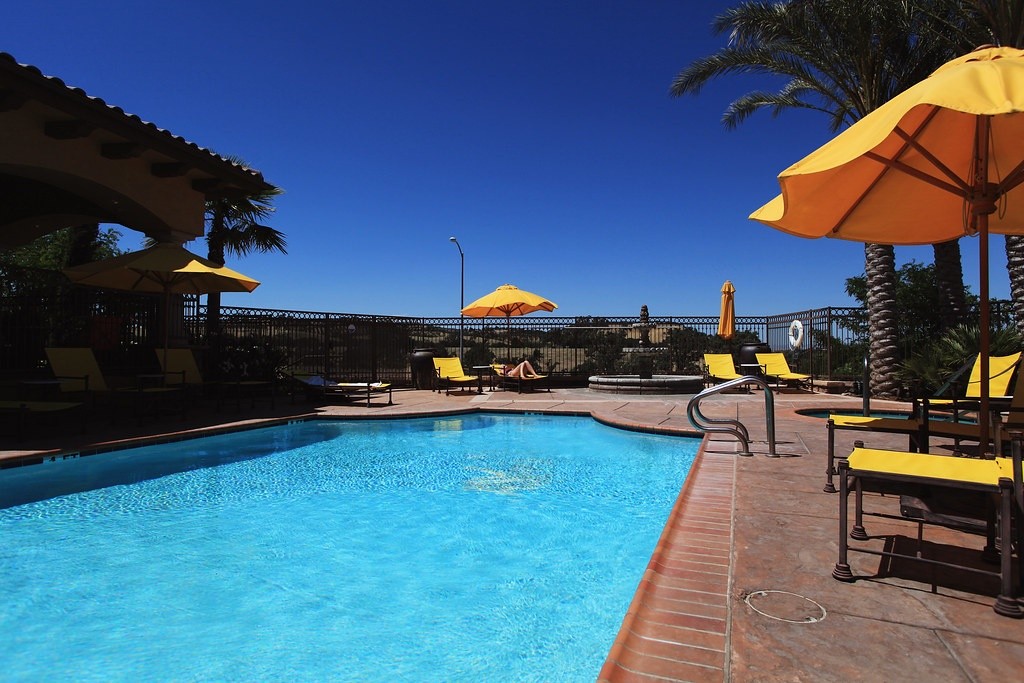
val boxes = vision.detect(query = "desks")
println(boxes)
[739,363,767,390]
[136,370,185,417]
[470,365,497,391]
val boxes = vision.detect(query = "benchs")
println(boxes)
[489,362,552,394]
[918,351,1022,457]
[44,346,185,425]
[755,352,815,395]
[156,347,276,416]
[431,357,483,396]
[0,384,88,443]
[704,353,754,394]
[291,371,393,408]
[838,441,1024,619]
[824,356,1024,493]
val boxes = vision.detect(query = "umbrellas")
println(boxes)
[460,283,558,363]
[62,242,262,388]
[717,280,736,353]
[746,45,1024,457]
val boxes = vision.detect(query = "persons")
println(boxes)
[498,361,542,379]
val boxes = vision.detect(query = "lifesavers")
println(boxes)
[788,320,803,347]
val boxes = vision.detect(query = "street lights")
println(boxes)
[449,236,464,366]
